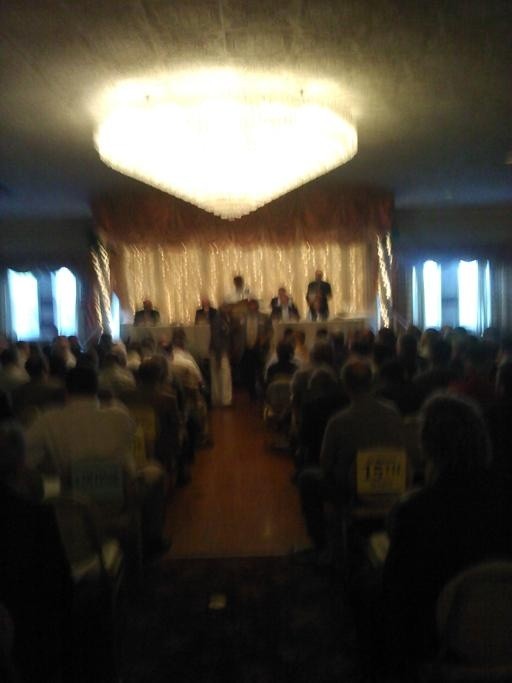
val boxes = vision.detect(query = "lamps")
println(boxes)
[93,66,358,224]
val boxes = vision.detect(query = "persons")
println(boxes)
[270,296,300,321]
[194,299,210,325]
[226,301,273,407]
[222,276,255,316]
[270,289,285,309]
[134,301,159,324]
[305,271,332,320]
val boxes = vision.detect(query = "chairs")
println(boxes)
[336,441,414,570]
[435,562,511,681]
[69,451,147,565]
[37,492,130,681]
